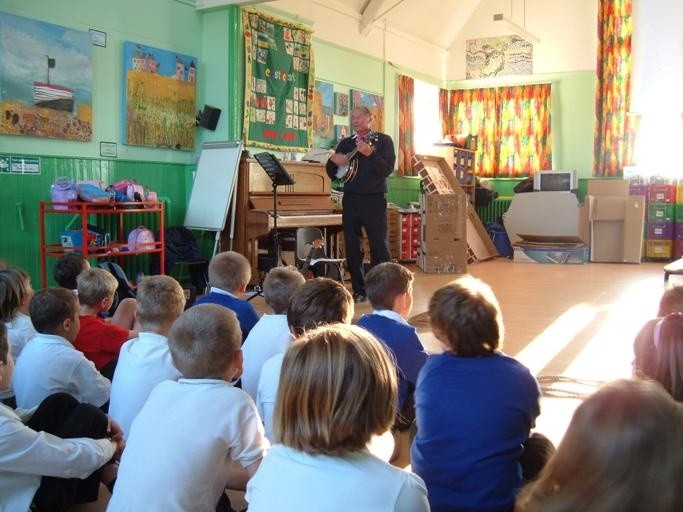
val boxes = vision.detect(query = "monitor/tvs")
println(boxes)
[533,169,578,192]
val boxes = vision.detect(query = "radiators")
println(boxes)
[474,196,514,224]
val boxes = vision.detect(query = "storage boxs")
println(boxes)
[645,183,683,259]
[584,180,645,265]
[425,193,467,273]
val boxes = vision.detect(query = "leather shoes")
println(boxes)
[352,291,367,304]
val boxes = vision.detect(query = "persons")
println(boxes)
[355,261,425,432]
[105,303,269,512]
[241,267,306,399]
[632,313,681,397]
[53,253,90,290]
[0,318,127,511]
[410,274,557,511]
[327,105,396,306]
[191,251,260,341]
[1,266,39,363]
[74,267,140,379]
[520,379,683,512]
[245,323,428,511]
[12,289,112,409]
[109,275,186,436]
[258,277,355,446]
[657,287,682,315]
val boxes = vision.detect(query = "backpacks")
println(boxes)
[99,262,136,316]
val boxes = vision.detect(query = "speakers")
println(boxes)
[195,104,221,131]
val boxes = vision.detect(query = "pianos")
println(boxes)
[218,158,346,286]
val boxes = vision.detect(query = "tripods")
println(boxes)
[244,183,279,301]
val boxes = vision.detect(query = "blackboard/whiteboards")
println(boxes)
[183,141,244,231]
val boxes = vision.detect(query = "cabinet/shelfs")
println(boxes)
[432,145,475,210]
[35,196,170,290]
